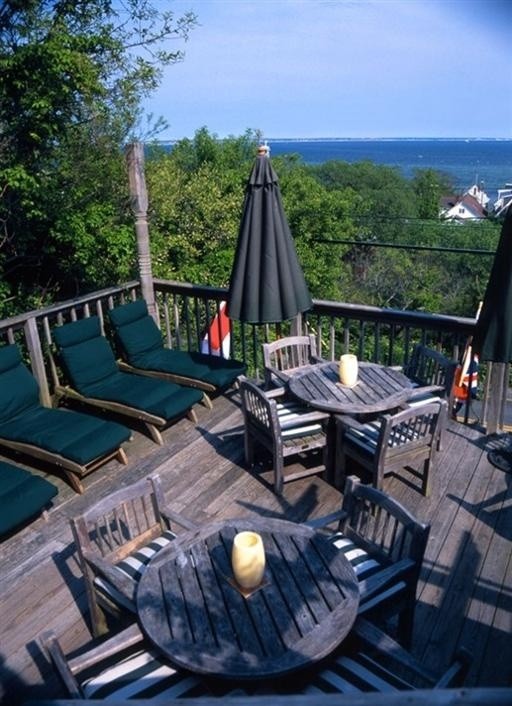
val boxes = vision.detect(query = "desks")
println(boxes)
[288,360,413,468]
[137,515,360,697]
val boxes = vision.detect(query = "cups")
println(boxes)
[338,353,358,387]
[231,530,266,590]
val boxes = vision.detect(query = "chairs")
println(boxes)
[69,472,200,632]
[37,621,247,699]
[298,474,432,651]
[283,616,481,697]
[333,396,447,497]
[108,300,249,410]
[238,378,332,496]
[45,314,204,446]
[0,460,58,539]
[394,341,461,452]
[262,332,328,401]
[0,344,134,496]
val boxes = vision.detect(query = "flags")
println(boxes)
[200,297,232,363]
[451,335,480,414]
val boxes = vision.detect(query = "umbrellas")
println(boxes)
[221,140,321,343]
[469,203,511,366]
